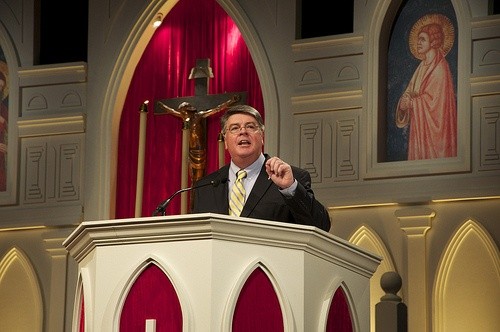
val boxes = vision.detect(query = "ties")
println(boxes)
[228,170,247,218]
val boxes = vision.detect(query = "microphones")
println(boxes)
[221,178,231,185]
[159,178,219,216]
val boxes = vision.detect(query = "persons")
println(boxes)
[156,94,241,215]
[190,103,333,233]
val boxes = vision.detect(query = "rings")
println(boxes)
[281,159,284,164]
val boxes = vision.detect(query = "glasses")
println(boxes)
[225,124,262,134]
